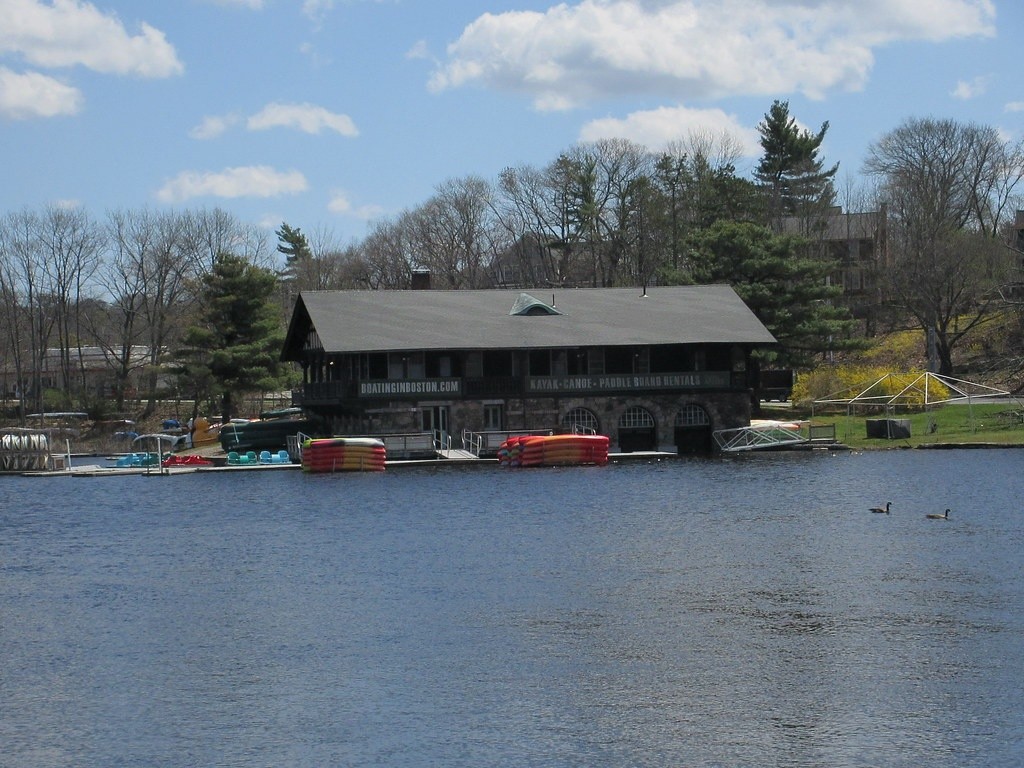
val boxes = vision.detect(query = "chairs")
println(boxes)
[230,451,256,463]
[260,451,289,463]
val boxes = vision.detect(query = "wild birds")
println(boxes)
[926,509,950,519]
[868,501,892,513]
